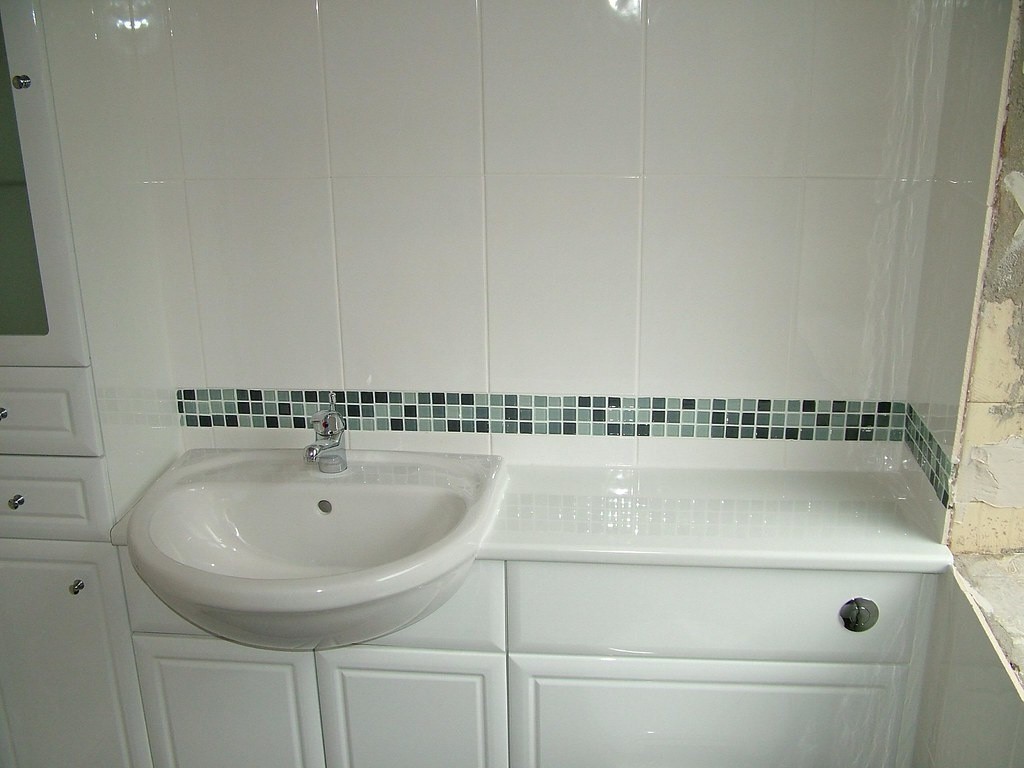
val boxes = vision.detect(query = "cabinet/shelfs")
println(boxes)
[0,0,178,768]
[504,559,938,767]
[118,545,509,768]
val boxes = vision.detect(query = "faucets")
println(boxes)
[303,411,348,473]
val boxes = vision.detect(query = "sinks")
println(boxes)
[127,448,505,651]
